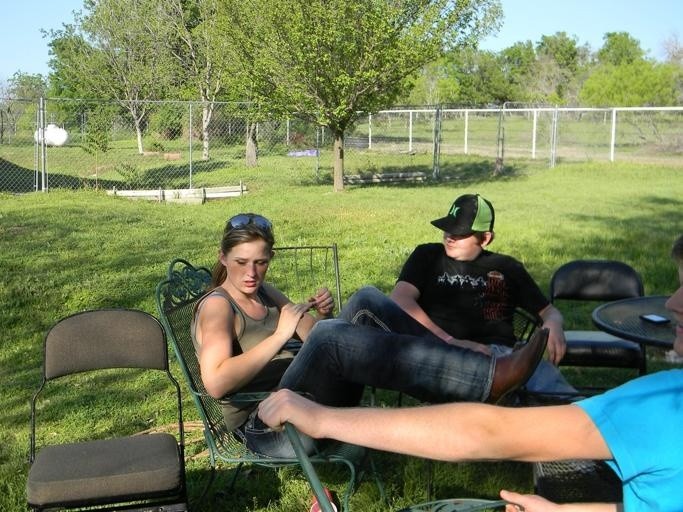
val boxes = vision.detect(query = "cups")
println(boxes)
[309,486,338,512]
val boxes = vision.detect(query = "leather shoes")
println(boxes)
[486,326,552,405]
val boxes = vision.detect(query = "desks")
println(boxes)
[591,296,678,377]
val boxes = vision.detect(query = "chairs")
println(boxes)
[157,258,388,511]
[546,258,647,399]
[24,308,188,511]
[263,243,376,409]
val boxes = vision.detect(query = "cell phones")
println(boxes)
[639,312,671,325]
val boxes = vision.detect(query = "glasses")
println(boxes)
[222,214,273,241]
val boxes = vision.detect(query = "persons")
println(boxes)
[189,212,552,461]
[256,232,683,511]
[384,193,589,406]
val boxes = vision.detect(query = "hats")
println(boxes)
[430,193,494,233]
